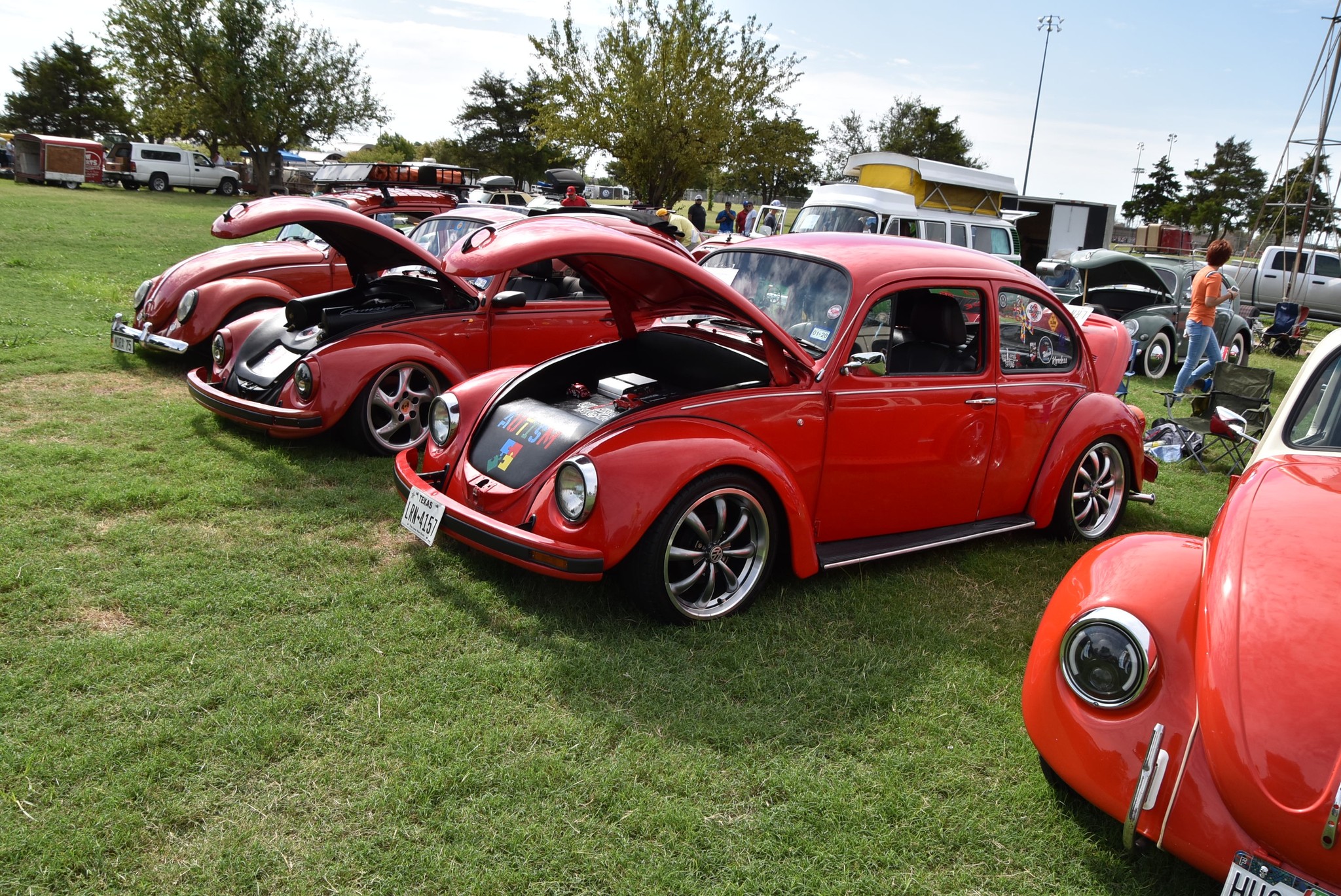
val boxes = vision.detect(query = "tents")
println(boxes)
[239,147,306,162]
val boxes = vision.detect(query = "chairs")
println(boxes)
[1249,301,1311,359]
[1152,360,1273,477]
[883,294,979,374]
[504,258,559,301]
[1239,304,1261,329]
[567,272,604,299]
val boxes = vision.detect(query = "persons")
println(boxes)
[5,138,14,167]
[655,208,707,252]
[688,195,707,232]
[744,202,757,237]
[211,151,225,165]
[102,147,108,160]
[735,201,749,234]
[561,186,586,206]
[225,156,232,165]
[715,201,736,234]
[763,199,781,235]
[1173,239,1238,402]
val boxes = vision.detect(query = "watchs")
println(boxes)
[750,229,752,232]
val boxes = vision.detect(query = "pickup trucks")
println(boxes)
[1196,246,1341,327]
[104,141,241,197]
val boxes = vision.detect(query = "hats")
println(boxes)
[566,186,575,195]
[747,202,754,209]
[743,201,749,206]
[656,208,669,217]
[771,199,785,207]
[725,201,731,206]
[696,195,702,200]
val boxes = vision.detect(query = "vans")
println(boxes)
[750,184,1022,276]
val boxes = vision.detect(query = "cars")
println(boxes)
[691,234,753,262]
[186,197,710,460]
[461,175,533,207]
[1067,248,1252,382]
[392,213,1159,626]
[1023,323,1340,896]
[111,188,460,370]
[526,168,590,208]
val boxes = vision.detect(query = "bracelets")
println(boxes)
[1229,292,1233,299]
